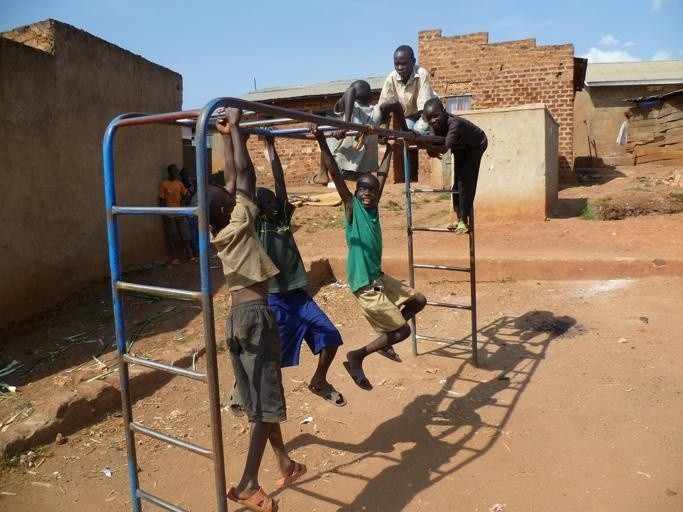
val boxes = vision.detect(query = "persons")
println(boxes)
[309,78,420,186]
[376,43,438,136]
[228,125,346,416]
[189,104,306,512]
[310,124,427,391]
[158,164,196,267]
[424,99,488,234]
[179,167,197,261]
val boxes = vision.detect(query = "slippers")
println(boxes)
[309,384,346,407]
[230,381,245,417]
[343,362,372,390]
[277,461,307,484]
[448,219,469,235]
[227,486,278,512]
[305,175,325,186]
[377,348,401,362]
